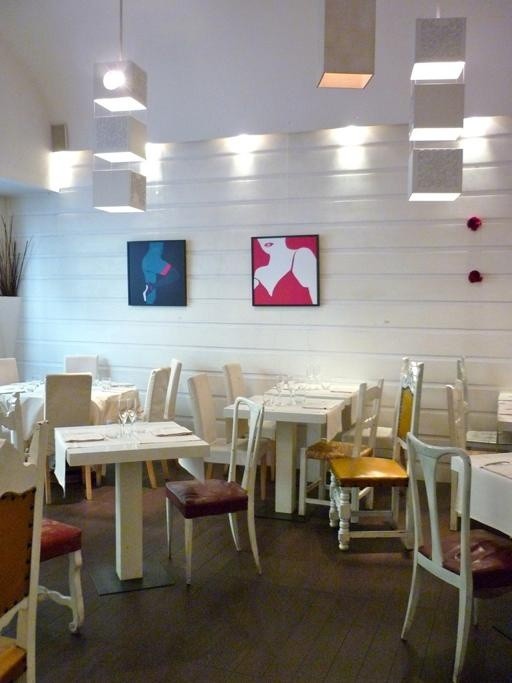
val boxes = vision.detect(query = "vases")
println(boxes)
[0,296,22,358]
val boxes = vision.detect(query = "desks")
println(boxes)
[2,376,142,499]
[448,452,511,629]
[222,378,362,522]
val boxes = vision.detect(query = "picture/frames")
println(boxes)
[126,239,187,307]
[251,234,320,306]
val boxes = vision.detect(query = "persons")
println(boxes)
[141,241,180,306]
[253,238,317,304]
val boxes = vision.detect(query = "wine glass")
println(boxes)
[267,365,323,405]
[117,392,138,439]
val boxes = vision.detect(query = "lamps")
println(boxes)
[406,0,465,203]
[91,0,148,214]
[315,0,374,90]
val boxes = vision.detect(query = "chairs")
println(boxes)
[95,365,170,488]
[62,355,109,477]
[0,418,50,683]
[163,396,266,587]
[1,357,20,385]
[39,515,84,634]
[446,357,512,533]
[328,354,425,552]
[188,371,269,499]
[221,360,277,486]
[44,372,94,506]
[400,433,511,682]
[163,359,183,420]
[297,376,388,518]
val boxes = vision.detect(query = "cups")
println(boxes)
[262,391,275,406]
[103,415,120,437]
